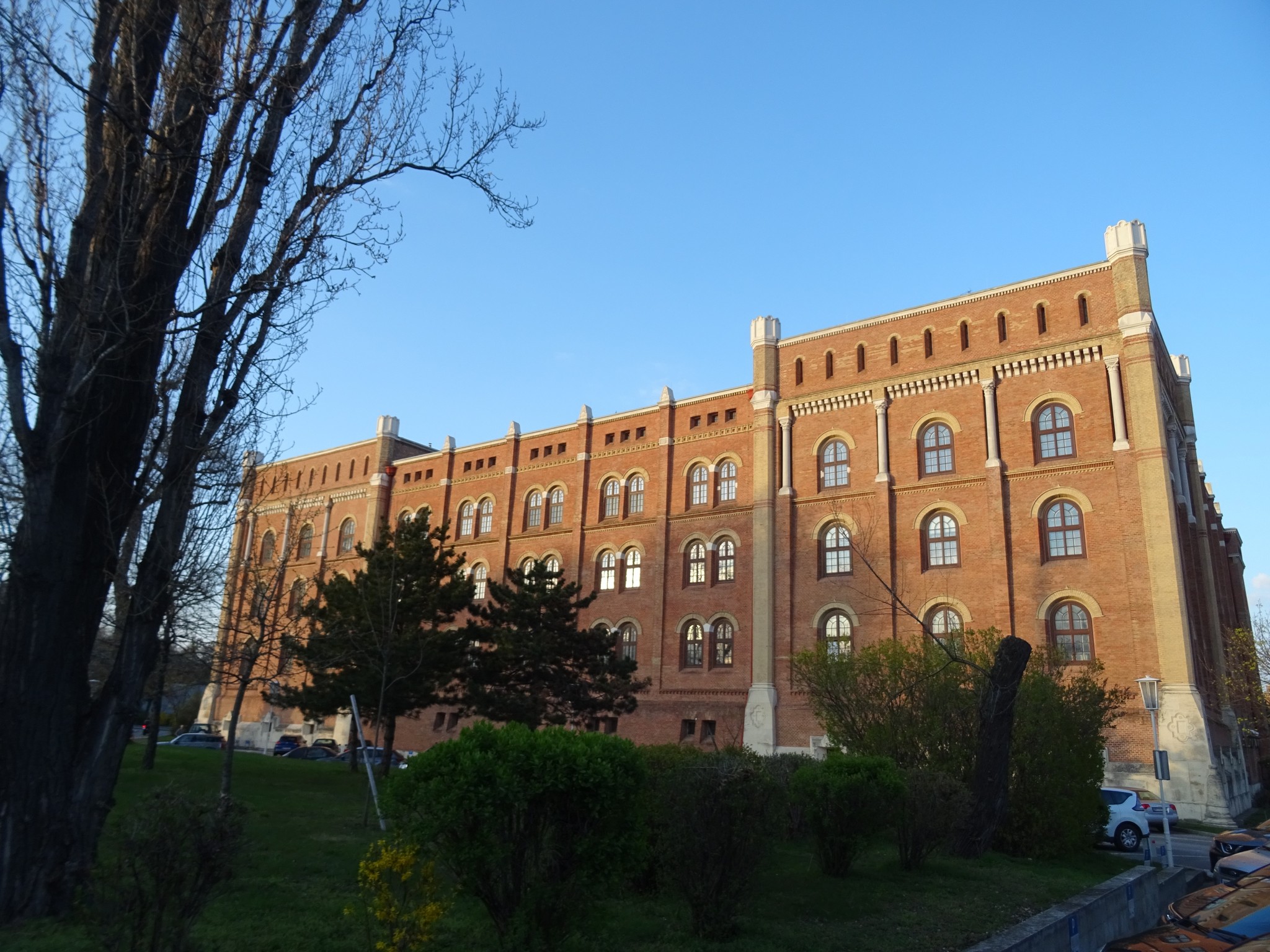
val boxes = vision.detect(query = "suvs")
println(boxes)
[155,732,225,750]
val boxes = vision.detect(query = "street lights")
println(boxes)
[1133,674,1173,869]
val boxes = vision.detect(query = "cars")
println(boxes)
[280,746,338,763]
[176,723,221,736]
[344,738,374,754]
[1099,786,1149,851]
[312,738,341,753]
[315,747,406,769]
[1095,819,1270,952]
[271,734,307,757]
[1103,786,1179,831]
